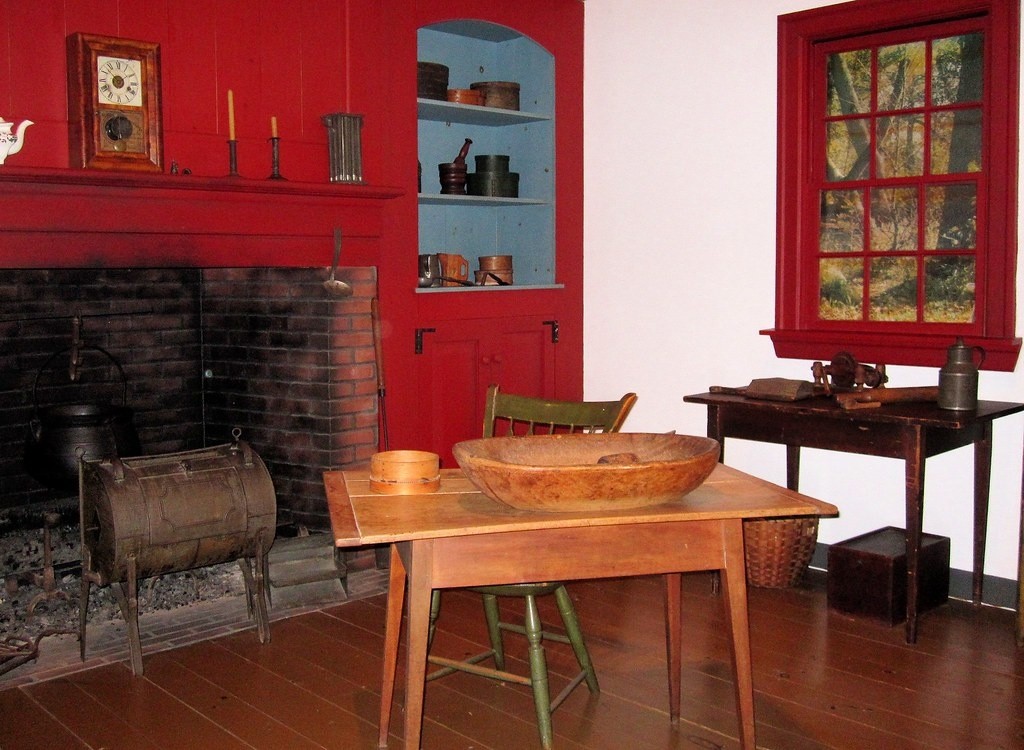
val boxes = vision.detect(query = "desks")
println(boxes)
[683,384,1024,646]
[323,458,840,750]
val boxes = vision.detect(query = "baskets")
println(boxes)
[743,516,820,589]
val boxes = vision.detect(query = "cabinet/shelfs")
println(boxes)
[407,1,565,293]
[405,314,560,471]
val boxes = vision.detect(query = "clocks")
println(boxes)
[66,32,165,172]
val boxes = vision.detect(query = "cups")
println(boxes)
[437,164,469,194]
[418,255,438,286]
[438,253,469,286]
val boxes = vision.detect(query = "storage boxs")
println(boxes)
[827,526,951,629]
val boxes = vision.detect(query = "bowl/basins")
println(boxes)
[451,431,719,510]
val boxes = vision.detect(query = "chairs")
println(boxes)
[426,385,639,749]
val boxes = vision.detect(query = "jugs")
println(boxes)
[939,337,984,413]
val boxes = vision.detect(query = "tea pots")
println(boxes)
[0,115,36,166]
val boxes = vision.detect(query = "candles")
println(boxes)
[270,116,278,137]
[228,89,236,140]
